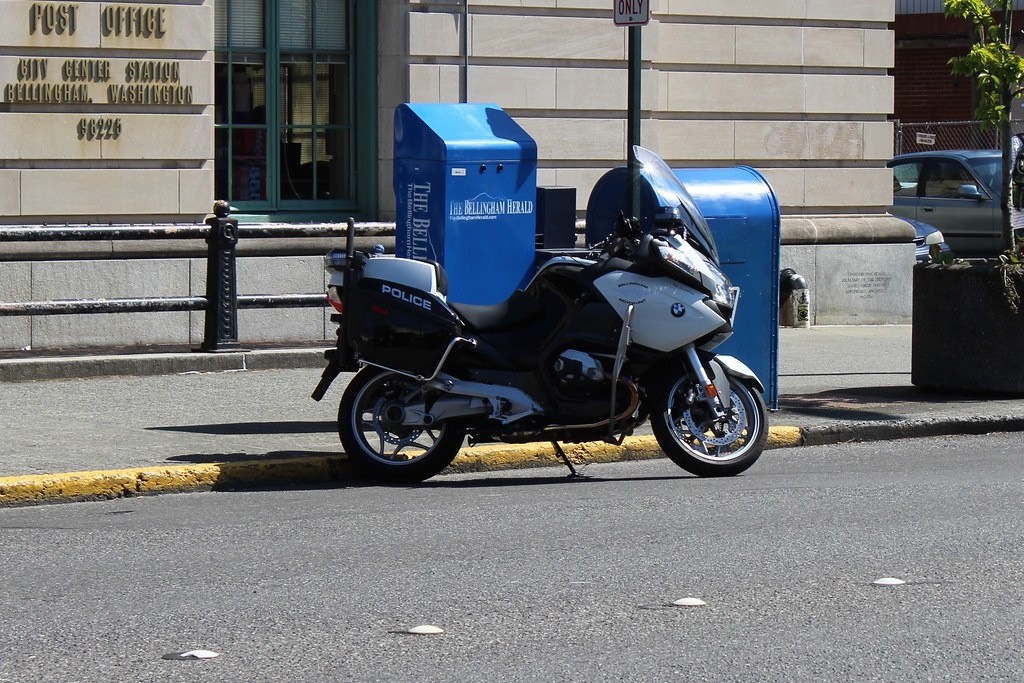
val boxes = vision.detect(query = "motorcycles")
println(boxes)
[310,145,769,485]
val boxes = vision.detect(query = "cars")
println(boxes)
[892,214,952,264]
[887,140,1024,257]
